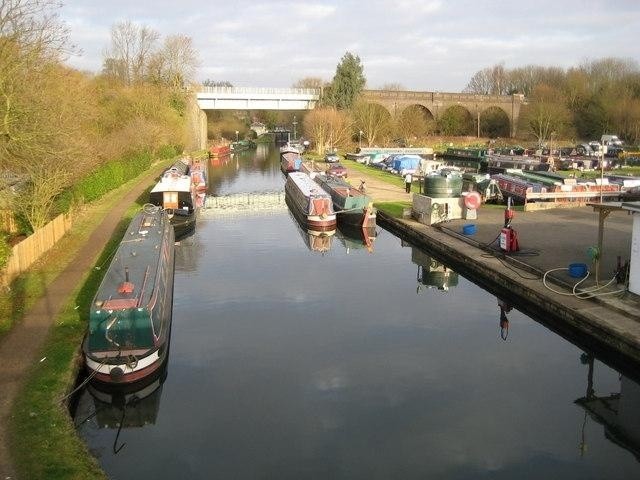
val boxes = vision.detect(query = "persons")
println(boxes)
[357,180,367,195]
[404,173,413,193]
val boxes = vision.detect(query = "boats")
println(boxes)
[280,153,304,175]
[80,371,171,428]
[334,220,378,254]
[80,202,176,384]
[167,138,259,175]
[149,172,196,243]
[298,160,326,179]
[313,175,377,228]
[287,208,333,256]
[344,134,640,203]
[280,132,310,162]
[284,169,337,227]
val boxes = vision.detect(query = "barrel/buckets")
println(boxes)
[463,224,477,235]
[568,262,588,278]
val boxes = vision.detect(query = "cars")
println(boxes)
[324,153,340,163]
[324,163,348,178]
